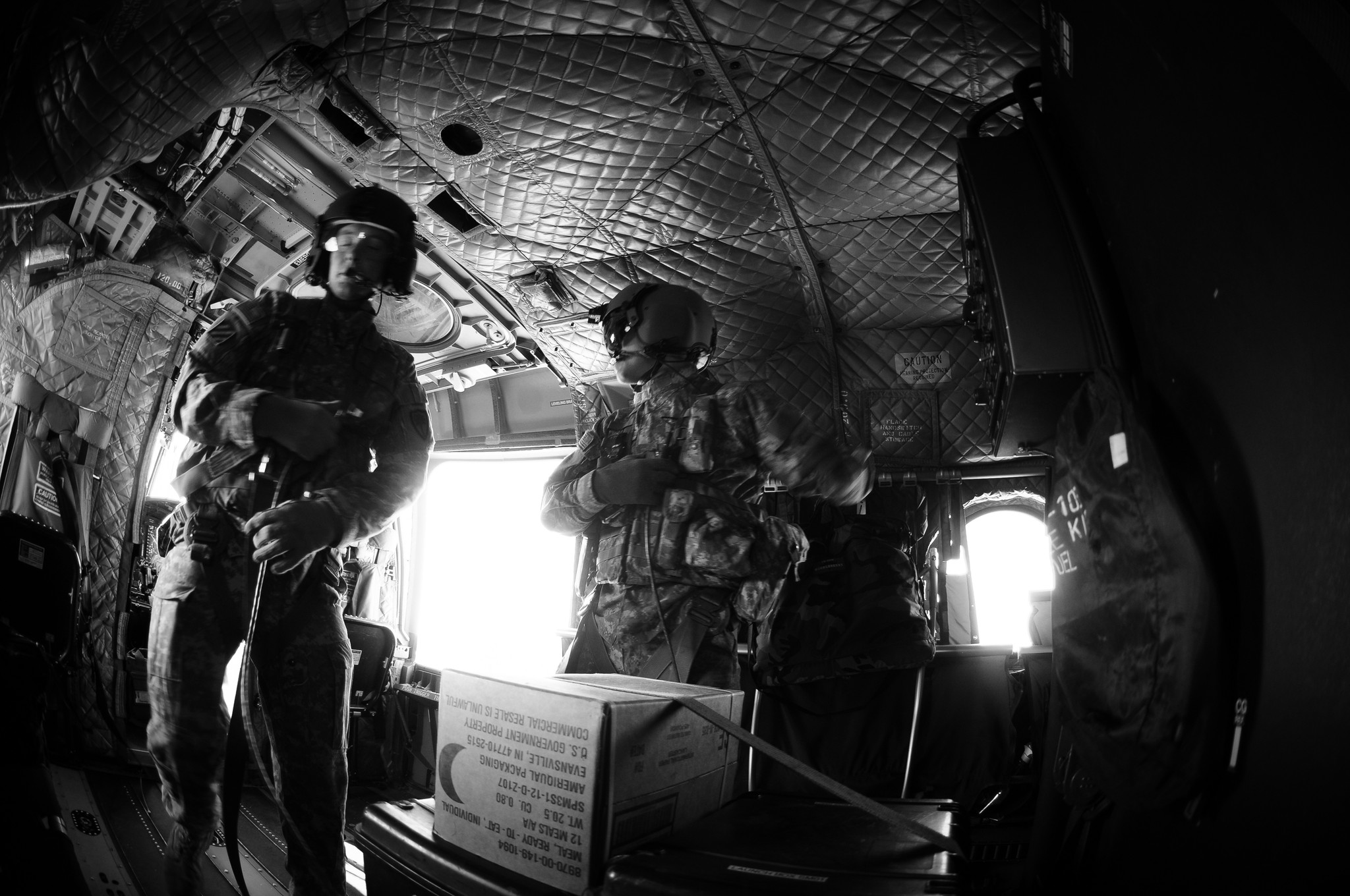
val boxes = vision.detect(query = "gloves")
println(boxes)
[240,493,346,575]
[819,447,871,506]
[255,391,347,463]
[593,451,680,507]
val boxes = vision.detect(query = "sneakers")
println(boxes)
[166,856,204,896]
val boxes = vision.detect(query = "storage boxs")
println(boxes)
[352,798,567,896]
[424,666,757,896]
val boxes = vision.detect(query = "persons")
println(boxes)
[539,275,876,690]
[148,187,434,896]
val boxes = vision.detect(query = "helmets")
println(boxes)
[306,187,419,302]
[604,282,718,356]
